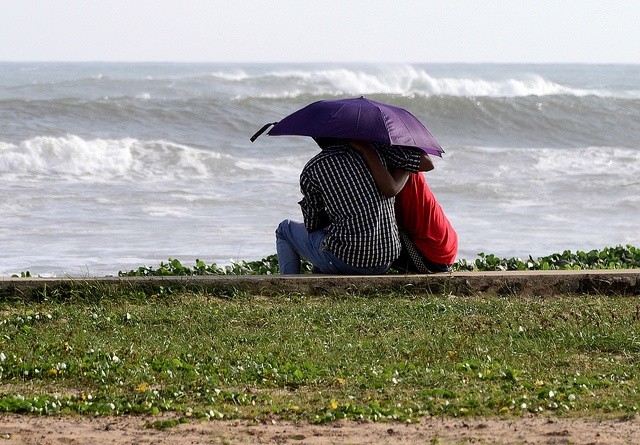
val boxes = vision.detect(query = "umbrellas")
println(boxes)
[250,95,445,158]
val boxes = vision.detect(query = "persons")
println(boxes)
[275,136,434,275]
[348,141,458,273]
